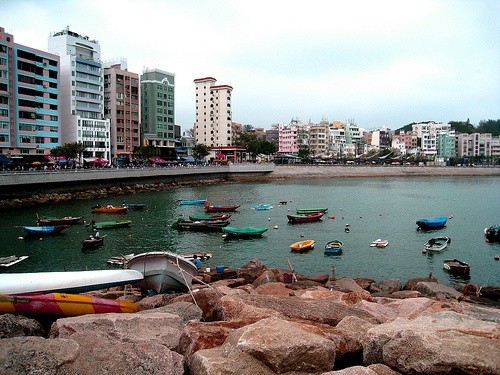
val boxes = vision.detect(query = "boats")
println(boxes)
[84,219,133,229]
[288,239,315,251]
[81,232,107,248]
[94,206,130,213]
[286,207,329,223]
[483,224,500,242]
[14,215,83,237]
[175,213,233,232]
[422,235,451,253]
[416,215,447,230]
[106,251,212,295]
[204,202,240,212]
[443,259,471,275]
[323,239,344,256]
[220,227,269,236]
[177,198,207,205]
[119,203,145,210]
[0,269,145,319]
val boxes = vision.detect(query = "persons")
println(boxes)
[192,254,202,270]
[53,164,65,173]
[95,230,100,237]
[44,165,48,172]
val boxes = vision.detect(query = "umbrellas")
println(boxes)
[47,161,57,166]
[32,161,41,167]
